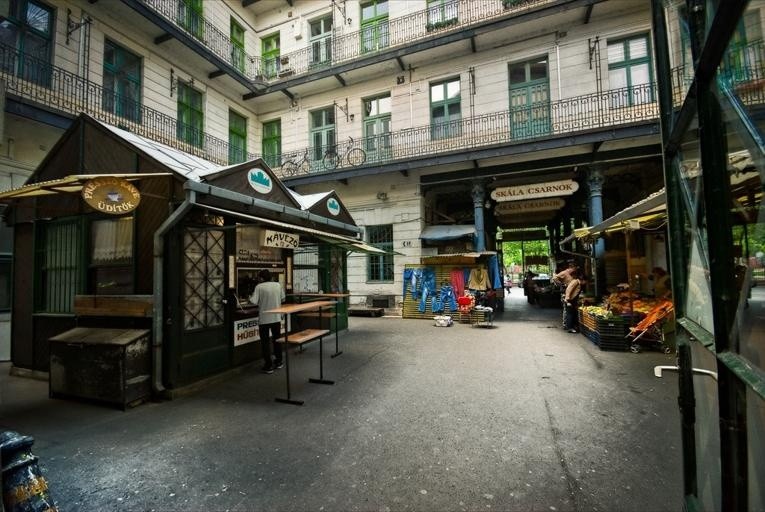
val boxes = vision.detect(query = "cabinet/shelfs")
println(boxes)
[48,327,151,412]
[262,300,339,406]
[288,293,351,358]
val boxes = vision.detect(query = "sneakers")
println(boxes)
[261,367,273,374]
[568,329,576,333]
[275,363,283,369]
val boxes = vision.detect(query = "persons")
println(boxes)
[560,274,590,334]
[548,259,578,308]
[246,266,289,374]
[636,266,671,300]
[504,274,512,294]
